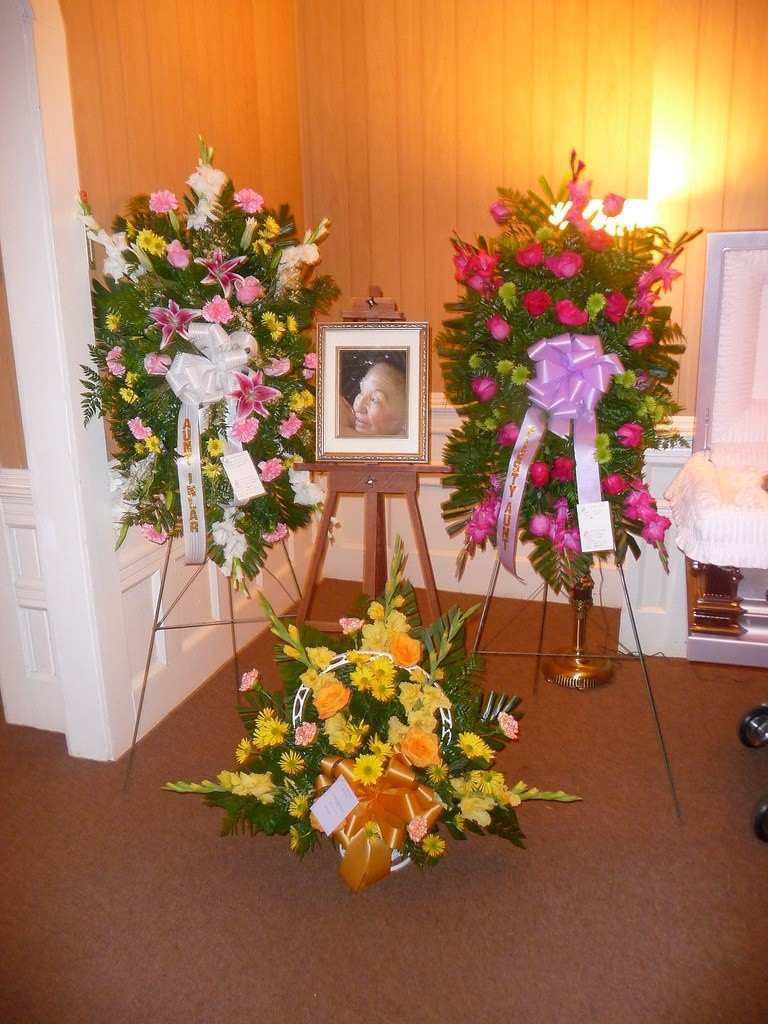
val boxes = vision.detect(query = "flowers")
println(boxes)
[435,133,699,595]
[68,133,332,594]
[172,558,580,875]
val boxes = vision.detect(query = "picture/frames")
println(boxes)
[315,320,429,471]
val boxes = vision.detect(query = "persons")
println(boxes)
[339,359,406,437]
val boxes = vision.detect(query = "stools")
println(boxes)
[288,460,453,655]
